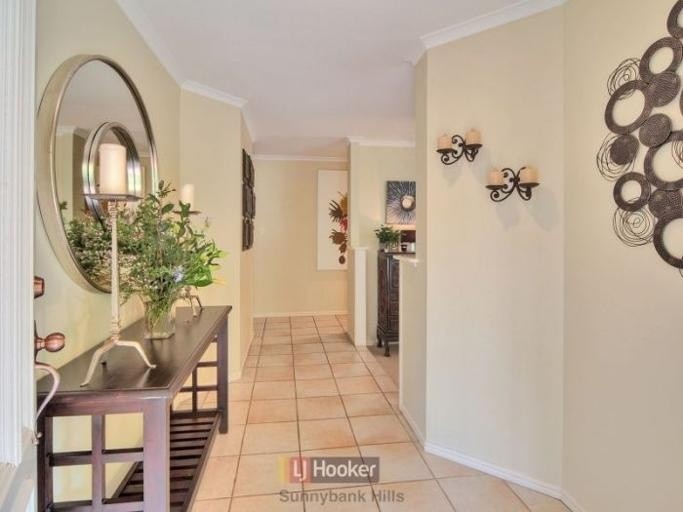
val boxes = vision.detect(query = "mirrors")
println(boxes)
[35,54,159,296]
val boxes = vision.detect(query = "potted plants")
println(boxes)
[374,224,401,253]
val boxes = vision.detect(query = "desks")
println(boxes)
[36,305,232,512]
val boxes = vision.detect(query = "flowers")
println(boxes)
[57,201,112,292]
[90,179,228,333]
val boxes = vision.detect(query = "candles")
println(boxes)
[519,167,539,183]
[437,134,452,152]
[465,128,480,146]
[487,167,504,185]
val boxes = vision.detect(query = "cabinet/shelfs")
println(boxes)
[376,249,399,357]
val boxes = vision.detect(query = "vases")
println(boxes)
[138,291,175,339]
